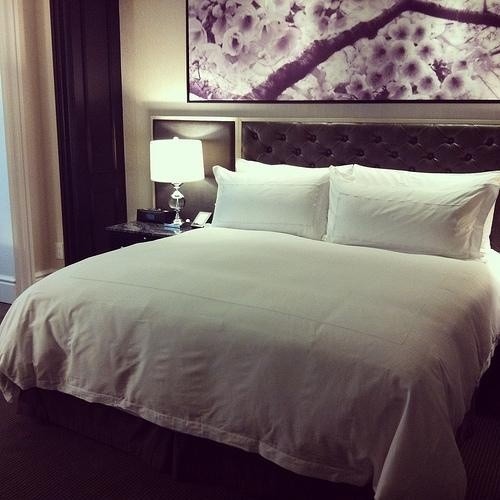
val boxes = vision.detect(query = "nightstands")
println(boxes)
[105,219,197,250]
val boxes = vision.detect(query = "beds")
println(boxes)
[0,115,500,500]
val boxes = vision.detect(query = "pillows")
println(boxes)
[321,164,500,261]
[339,164,500,190]
[234,156,335,179]
[210,165,328,242]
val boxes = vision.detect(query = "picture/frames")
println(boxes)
[190,211,212,227]
[186,0,500,106]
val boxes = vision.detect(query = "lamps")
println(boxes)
[148,135,205,227]
